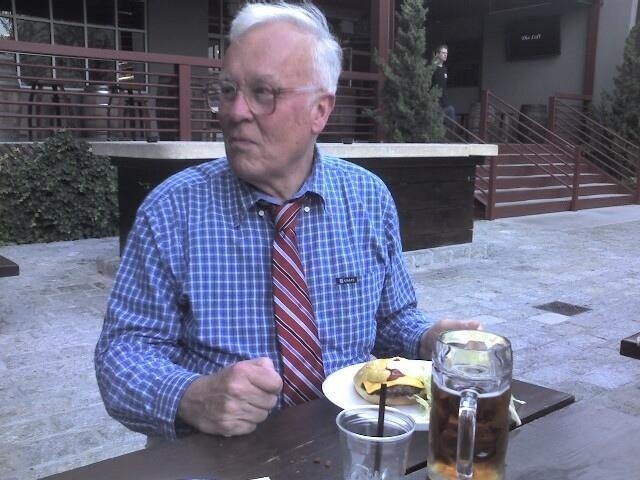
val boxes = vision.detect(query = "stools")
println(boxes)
[107,85,145,140]
[27,78,76,141]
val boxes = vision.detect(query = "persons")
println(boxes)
[428,43,459,127]
[91,1,483,446]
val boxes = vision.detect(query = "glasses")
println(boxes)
[205,78,325,118]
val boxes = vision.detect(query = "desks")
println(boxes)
[455,112,515,144]
[90,140,498,276]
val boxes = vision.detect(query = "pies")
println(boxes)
[354,358,427,406]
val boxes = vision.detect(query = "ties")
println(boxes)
[267,194,325,408]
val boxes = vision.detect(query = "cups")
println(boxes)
[334,405,415,480]
[426,328,513,480]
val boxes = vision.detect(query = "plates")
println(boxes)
[321,358,434,431]
